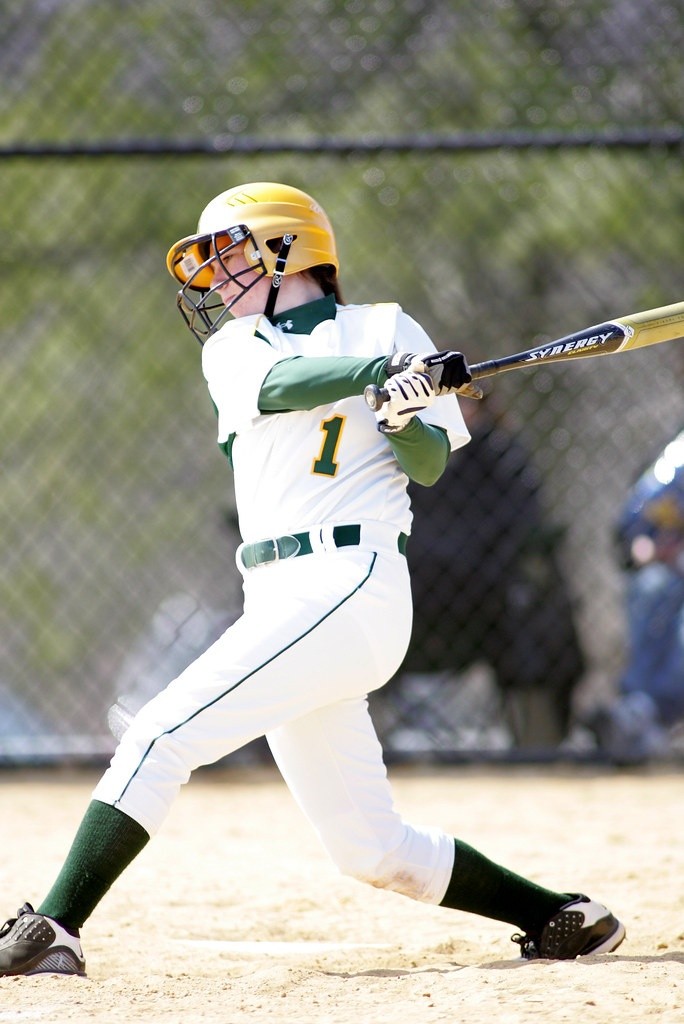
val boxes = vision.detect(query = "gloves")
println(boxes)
[386,350,473,396]
[375,361,435,433]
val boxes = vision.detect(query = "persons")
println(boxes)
[395,384,684,766]
[3,183,625,977]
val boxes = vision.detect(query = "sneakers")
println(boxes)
[0,902,87,977]
[511,893,626,960]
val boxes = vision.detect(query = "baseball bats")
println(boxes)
[363,300,684,420]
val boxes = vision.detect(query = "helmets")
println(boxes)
[166,182,339,346]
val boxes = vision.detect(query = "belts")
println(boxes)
[242,525,408,569]
